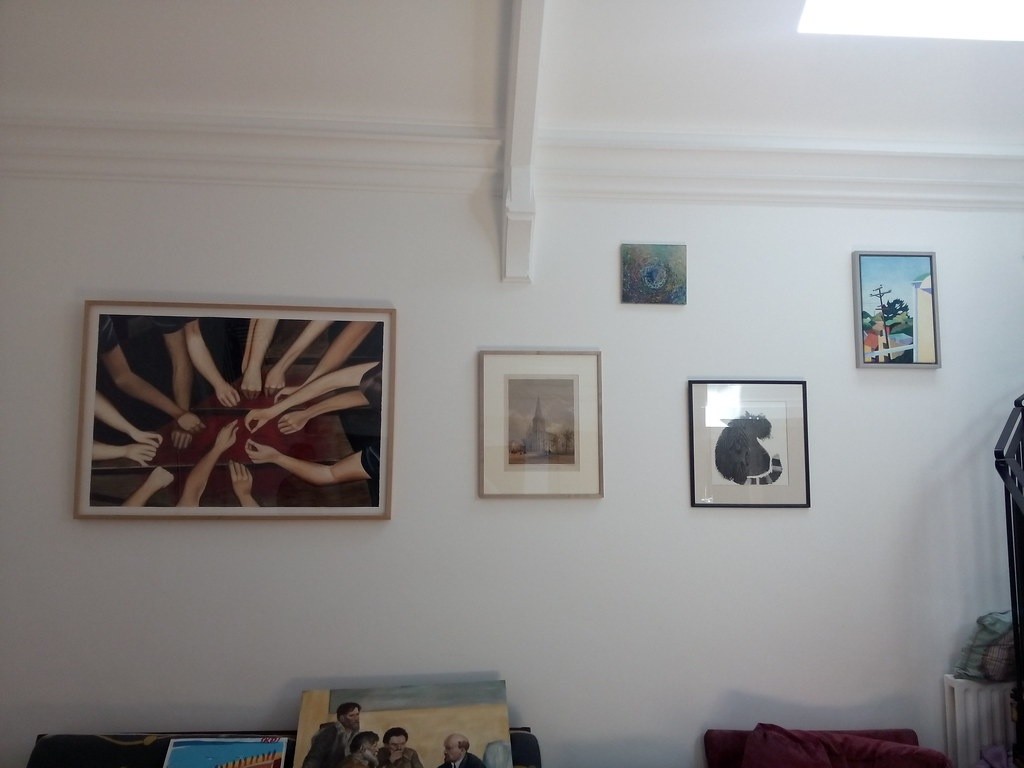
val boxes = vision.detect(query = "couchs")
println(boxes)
[704,723,948,768]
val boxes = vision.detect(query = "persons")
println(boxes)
[90,314,382,506]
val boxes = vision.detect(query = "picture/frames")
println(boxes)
[689,380,811,508]
[851,250,941,368]
[476,350,605,500]
[73,299,397,524]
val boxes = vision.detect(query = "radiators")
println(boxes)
[943,674,1017,768]
